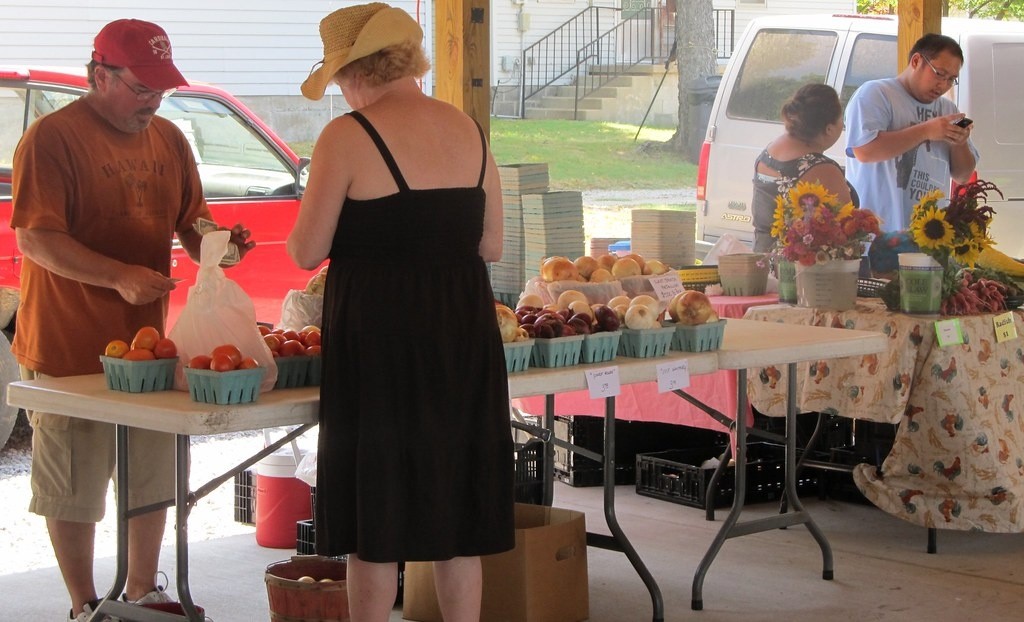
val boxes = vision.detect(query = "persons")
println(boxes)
[752,84,860,275]
[286,1,517,622]
[845,33,981,278]
[10,20,257,622]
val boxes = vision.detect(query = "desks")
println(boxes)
[521,291,1023,553]
[6,313,885,622]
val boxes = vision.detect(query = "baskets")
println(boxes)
[510,437,545,506]
[634,444,760,509]
[100,242,947,406]
[294,485,406,611]
[232,468,259,526]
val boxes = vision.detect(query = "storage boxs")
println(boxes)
[551,411,900,510]
[401,504,588,622]
[513,437,544,502]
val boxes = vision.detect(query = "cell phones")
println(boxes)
[954,118,974,129]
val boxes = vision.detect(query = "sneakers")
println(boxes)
[120,570,206,622]
[67,598,124,622]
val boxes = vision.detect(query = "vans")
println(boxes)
[693,12,1023,276]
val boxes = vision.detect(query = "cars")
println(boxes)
[0,64,333,440]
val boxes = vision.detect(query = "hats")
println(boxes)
[91,18,190,91]
[300,2,423,100]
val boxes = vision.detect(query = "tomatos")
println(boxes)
[105,326,178,362]
[188,343,259,372]
[255,323,322,356]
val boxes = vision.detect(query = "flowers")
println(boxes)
[911,171,1004,271]
[770,180,885,267]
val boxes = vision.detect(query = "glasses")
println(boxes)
[921,53,959,87]
[102,64,178,101]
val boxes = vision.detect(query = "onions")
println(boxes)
[494,252,720,345]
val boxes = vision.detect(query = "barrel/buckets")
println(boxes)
[265,554,350,622]
[256,427,313,549]
